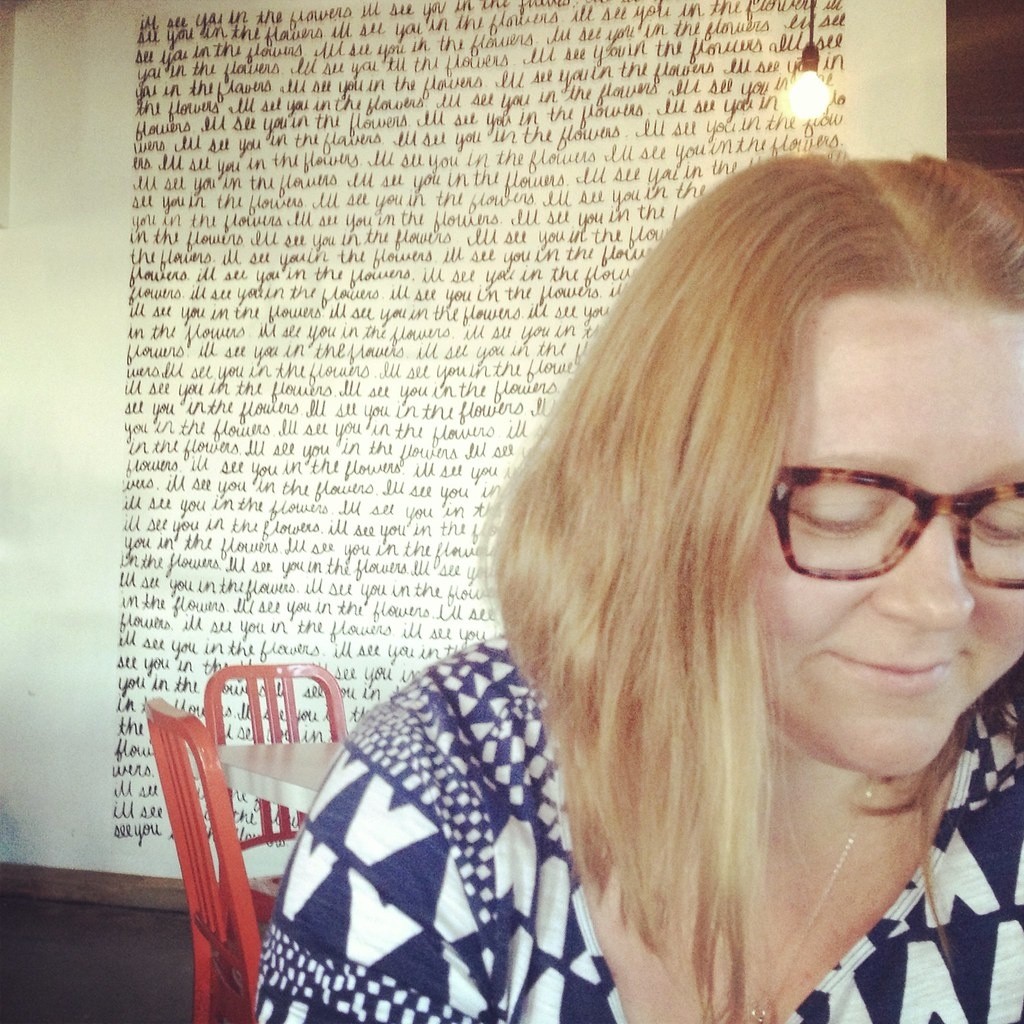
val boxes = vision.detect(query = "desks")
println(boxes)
[187,742,346,814]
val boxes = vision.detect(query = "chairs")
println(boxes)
[146,698,271,1024]
[205,665,348,922]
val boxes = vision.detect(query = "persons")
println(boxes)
[257,154,1023,1024]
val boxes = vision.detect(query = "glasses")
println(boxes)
[767,465,1024,590]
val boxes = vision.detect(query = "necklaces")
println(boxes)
[747,781,876,1024]
